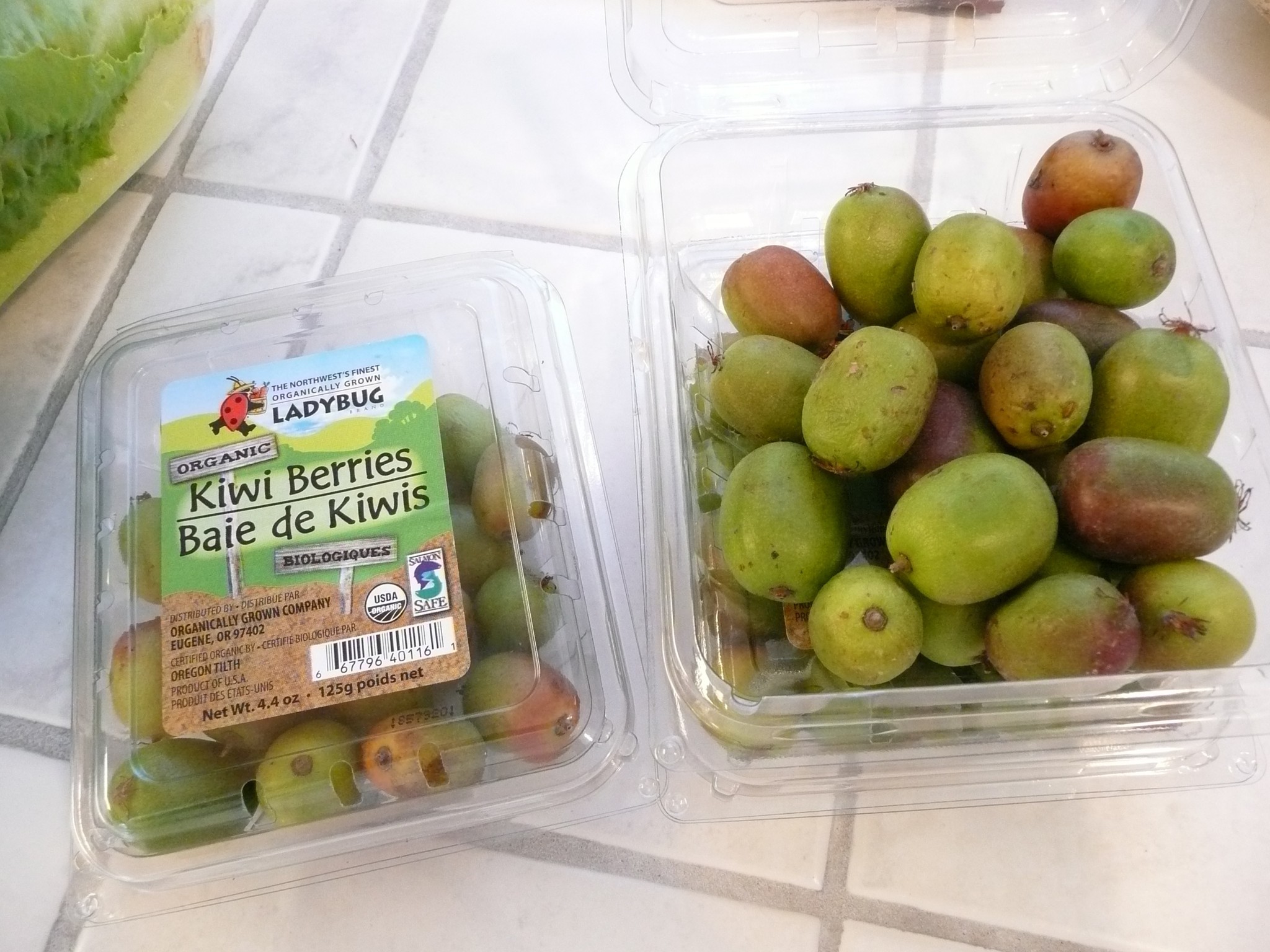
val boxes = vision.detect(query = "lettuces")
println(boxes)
[0,0,193,256]
